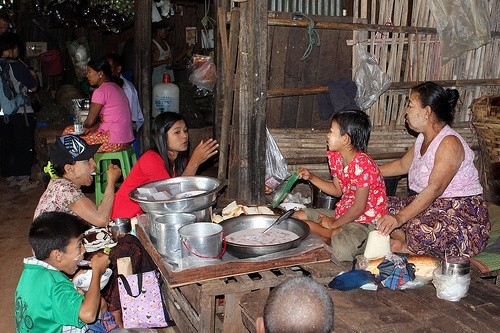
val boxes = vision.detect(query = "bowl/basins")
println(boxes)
[73,268,112,291]
[128,176,225,212]
[110,217,132,236]
[218,214,310,258]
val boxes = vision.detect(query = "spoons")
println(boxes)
[91,170,107,176]
[78,260,91,267]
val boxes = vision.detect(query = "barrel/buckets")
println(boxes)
[177,221,226,269]
[148,207,213,248]
[153,212,196,265]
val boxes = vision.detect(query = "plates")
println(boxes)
[70,131,84,135]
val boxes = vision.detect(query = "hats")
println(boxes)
[48,135,102,167]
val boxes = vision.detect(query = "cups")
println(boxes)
[74,124,83,132]
[441,256,471,278]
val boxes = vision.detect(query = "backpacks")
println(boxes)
[0,58,30,127]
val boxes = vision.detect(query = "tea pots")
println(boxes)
[77,99,90,109]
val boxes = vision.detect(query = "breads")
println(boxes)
[365,254,437,277]
[212,200,274,223]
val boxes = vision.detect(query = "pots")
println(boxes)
[310,184,339,211]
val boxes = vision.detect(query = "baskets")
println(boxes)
[468,94,500,163]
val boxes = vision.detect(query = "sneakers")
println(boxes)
[5,176,17,188]
[17,176,41,192]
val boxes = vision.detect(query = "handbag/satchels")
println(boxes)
[265,127,292,194]
[117,269,170,329]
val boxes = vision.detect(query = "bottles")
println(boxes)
[151,74,180,116]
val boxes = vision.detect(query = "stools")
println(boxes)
[94,147,137,206]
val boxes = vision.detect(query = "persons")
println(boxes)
[0,15,42,192]
[110,111,219,220]
[255,277,336,333]
[378,81,491,261]
[32,135,164,328]
[14,211,111,333]
[62,19,187,153]
[291,107,388,262]
